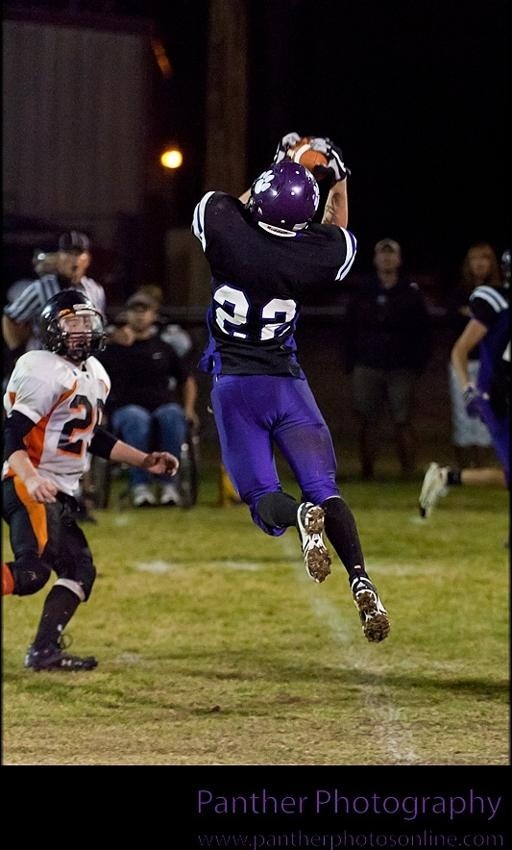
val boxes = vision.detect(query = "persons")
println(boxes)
[96,294,201,509]
[7,242,61,308]
[0,231,109,356]
[437,243,503,470]
[188,133,392,644]
[3,289,180,673]
[341,235,423,484]
[416,279,510,547]
[104,283,194,358]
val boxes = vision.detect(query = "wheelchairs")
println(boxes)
[84,410,208,516]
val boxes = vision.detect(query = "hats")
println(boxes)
[376,238,399,253]
[128,293,153,308]
[60,230,89,251]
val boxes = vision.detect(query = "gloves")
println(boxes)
[246,162,320,230]
[271,132,302,166]
[309,137,349,180]
[463,385,490,418]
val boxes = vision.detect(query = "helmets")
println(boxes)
[41,290,108,361]
[500,250,512,289]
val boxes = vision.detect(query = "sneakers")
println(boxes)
[297,503,331,583]
[133,484,157,505]
[24,643,98,670]
[349,572,390,643]
[159,483,180,504]
[419,462,448,517]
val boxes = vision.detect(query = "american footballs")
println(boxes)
[286,136,329,181]
